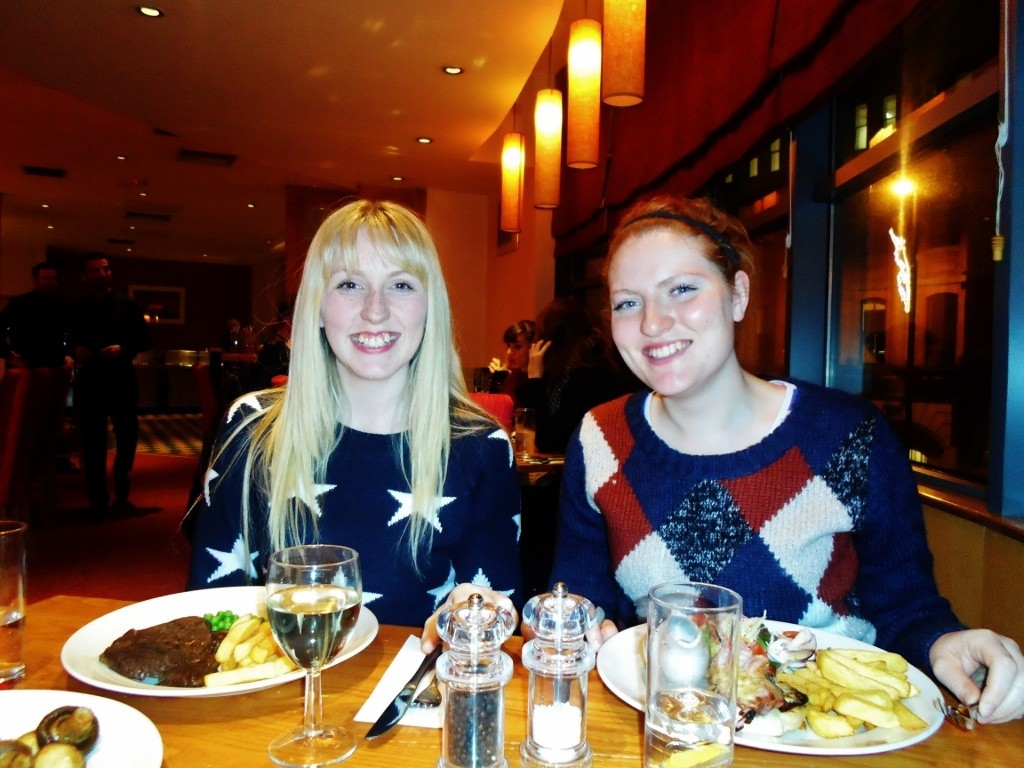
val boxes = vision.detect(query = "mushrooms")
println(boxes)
[0,705,100,768]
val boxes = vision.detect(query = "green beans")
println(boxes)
[204,610,241,632]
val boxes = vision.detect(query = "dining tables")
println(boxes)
[1,593,1024,768]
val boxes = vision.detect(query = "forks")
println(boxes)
[410,673,442,707]
[938,698,978,730]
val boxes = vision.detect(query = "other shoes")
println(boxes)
[114,500,135,514]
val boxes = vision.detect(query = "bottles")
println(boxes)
[436,593,515,768]
[521,582,595,768]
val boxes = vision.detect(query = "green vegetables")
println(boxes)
[702,619,782,670]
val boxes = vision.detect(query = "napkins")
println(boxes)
[353,633,445,728]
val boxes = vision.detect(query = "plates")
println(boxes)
[596,618,946,755]
[0,689,164,768]
[60,586,379,698]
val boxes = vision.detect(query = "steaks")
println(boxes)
[100,616,225,687]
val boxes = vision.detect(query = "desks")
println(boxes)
[514,452,566,471]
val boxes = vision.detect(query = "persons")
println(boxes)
[186,199,522,653]
[210,304,290,441]
[0,251,153,518]
[488,269,625,447]
[552,194,1023,727]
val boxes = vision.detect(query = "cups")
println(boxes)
[515,408,535,461]
[492,351,513,384]
[475,386,490,394]
[644,581,743,768]
[0,520,29,690]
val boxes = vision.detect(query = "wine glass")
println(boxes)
[265,544,363,768]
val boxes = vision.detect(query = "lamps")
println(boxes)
[501,102,526,232]
[567,0,601,169]
[603,0,650,106]
[535,37,564,209]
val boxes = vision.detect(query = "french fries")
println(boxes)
[780,648,928,737]
[202,614,299,688]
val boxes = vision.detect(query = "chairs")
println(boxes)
[0,345,259,522]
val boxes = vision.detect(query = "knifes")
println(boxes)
[365,641,443,740]
